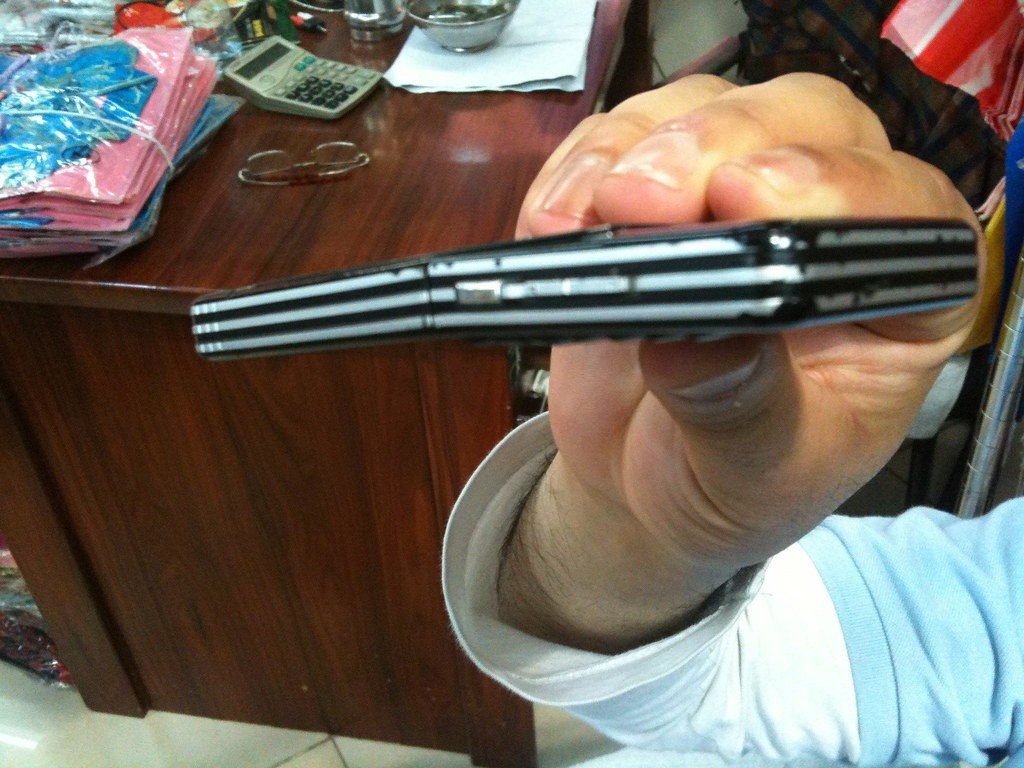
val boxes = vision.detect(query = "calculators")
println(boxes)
[223,34,385,120]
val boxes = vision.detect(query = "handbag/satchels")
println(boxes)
[736,0,1008,231]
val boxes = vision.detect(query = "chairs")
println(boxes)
[650,0,1023,518]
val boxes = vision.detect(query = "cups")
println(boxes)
[342,0,406,41]
[181,0,242,81]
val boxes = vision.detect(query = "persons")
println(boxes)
[440,73,1024,768]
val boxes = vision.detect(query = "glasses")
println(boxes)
[238,141,370,185]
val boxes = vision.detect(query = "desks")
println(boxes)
[0,0,650,768]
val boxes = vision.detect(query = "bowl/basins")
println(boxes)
[405,0,523,52]
